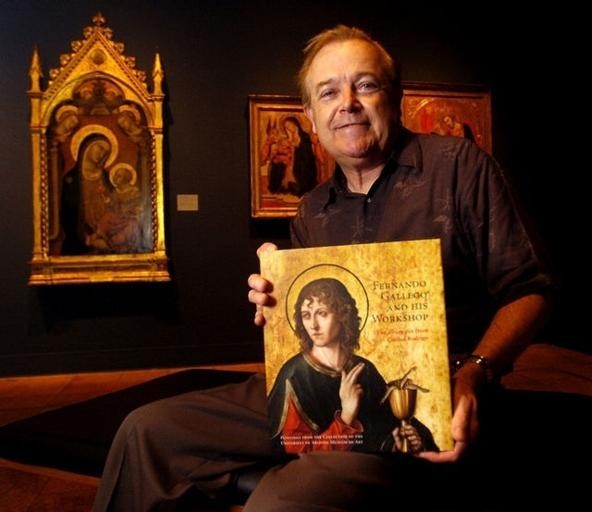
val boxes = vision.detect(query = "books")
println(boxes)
[256,237,460,462]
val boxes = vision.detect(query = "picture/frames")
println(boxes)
[248,95,336,218]
[398,78,494,156]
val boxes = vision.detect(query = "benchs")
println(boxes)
[0,369,592,512]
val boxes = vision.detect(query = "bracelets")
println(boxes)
[464,349,501,379]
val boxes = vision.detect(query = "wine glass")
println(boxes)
[387,377,417,454]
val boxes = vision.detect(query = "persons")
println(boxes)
[85,166,144,249]
[58,130,136,254]
[88,24,557,511]
[266,278,441,457]
[432,111,476,143]
[266,115,318,199]
